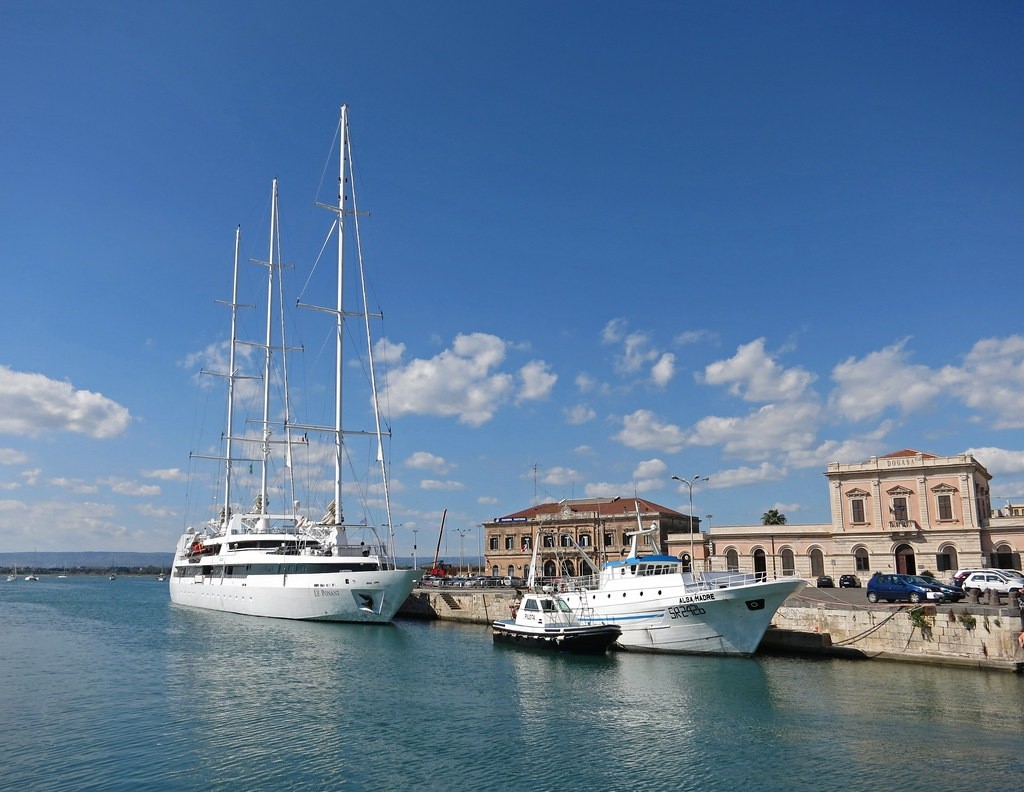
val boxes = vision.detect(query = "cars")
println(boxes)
[817,576,835,587]
[866,574,958,602]
[423,575,501,587]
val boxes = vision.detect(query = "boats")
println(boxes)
[513,500,801,654]
[491,527,626,656]
[110,574,116,580]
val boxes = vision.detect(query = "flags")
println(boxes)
[521,543,528,553]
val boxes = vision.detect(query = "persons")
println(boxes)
[361,542,371,557]
[1005,500,1013,518]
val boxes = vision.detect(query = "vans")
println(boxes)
[955,567,1024,595]
[504,576,524,585]
[840,574,861,587]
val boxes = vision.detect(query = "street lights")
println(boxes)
[671,475,710,570]
[412,529,419,571]
[476,524,483,575]
[381,524,403,567]
[451,527,471,576]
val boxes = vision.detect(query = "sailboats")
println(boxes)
[7,558,18,583]
[156,561,166,581]
[167,103,422,624]
[57,567,67,579]
[25,544,40,581]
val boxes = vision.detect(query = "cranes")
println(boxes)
[430,508,448,575]
